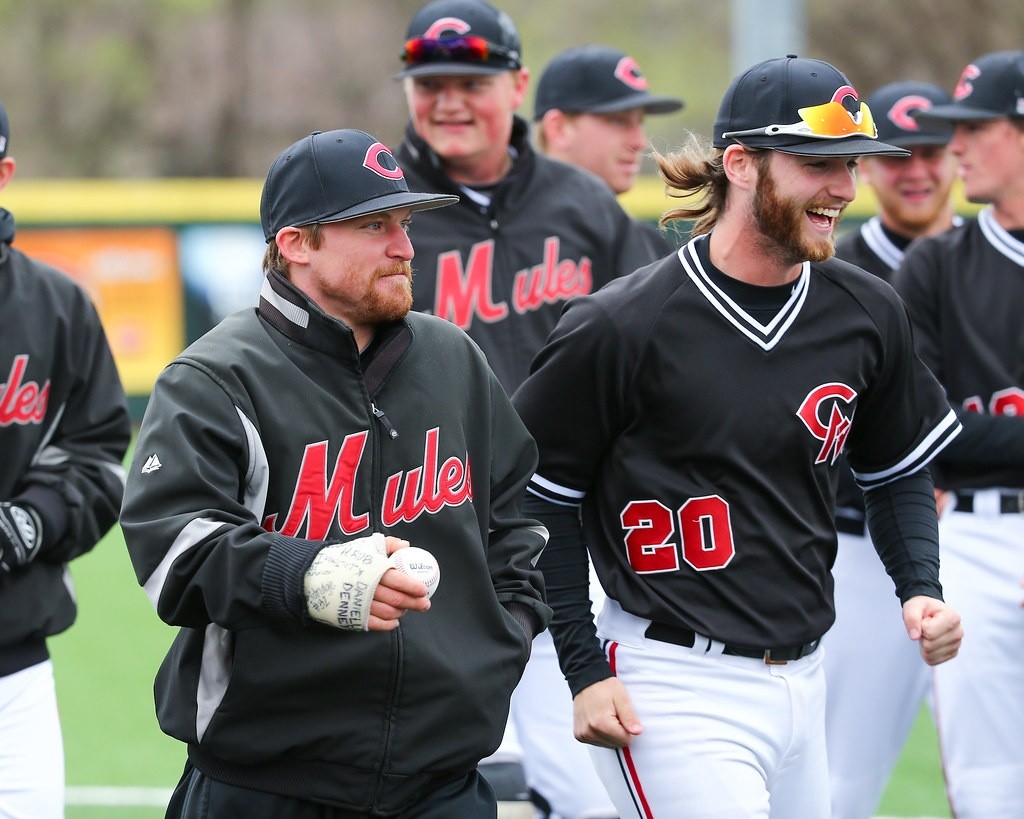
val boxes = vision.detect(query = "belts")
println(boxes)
[835,515,865,537]
[645,621,822,665]
[954,496,1024,514]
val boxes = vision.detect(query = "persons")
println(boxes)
[0,103,133,819]
[119,130,554,819]
[530,46,677,260]
[817,83,964,819]
[510,54,962,819]
[894,46,1024,819]
[384,0,658,819]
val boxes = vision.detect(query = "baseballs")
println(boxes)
[388,546,441,600]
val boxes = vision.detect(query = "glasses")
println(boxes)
[720,101,881,142]
[398,32,518,70]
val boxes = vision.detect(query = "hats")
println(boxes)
[260,128,461,245]
[867,79,954,148]
[712,54,914,159]
[911,51,1024,135]
[532,46,684,121]
[392,1,522,84]
